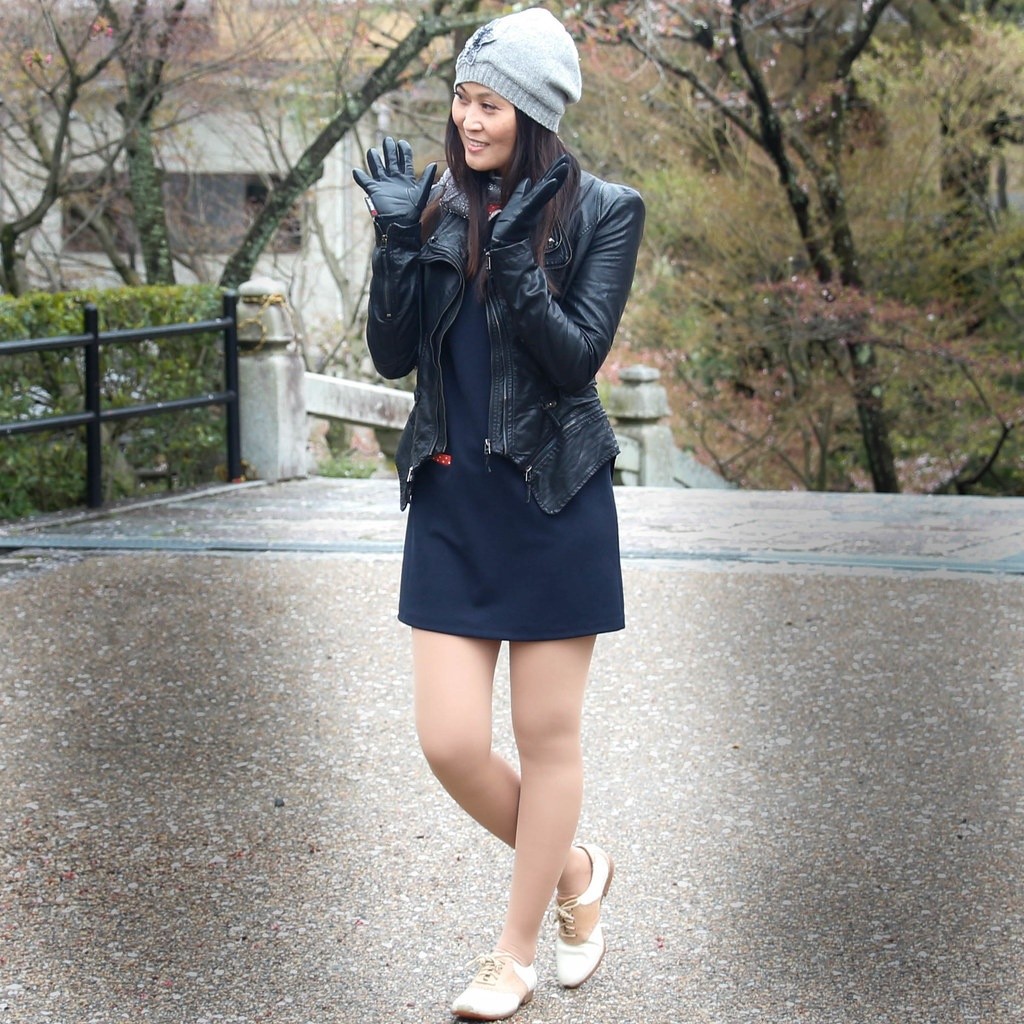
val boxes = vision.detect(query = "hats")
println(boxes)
[453,6,584,135]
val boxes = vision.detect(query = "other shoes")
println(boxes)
[548,840,616,990]
[450,950,538,1024]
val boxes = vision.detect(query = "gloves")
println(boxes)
[353,136,439,238]
[491,152,570,249]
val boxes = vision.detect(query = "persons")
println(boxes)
[351,5,650,1021]
